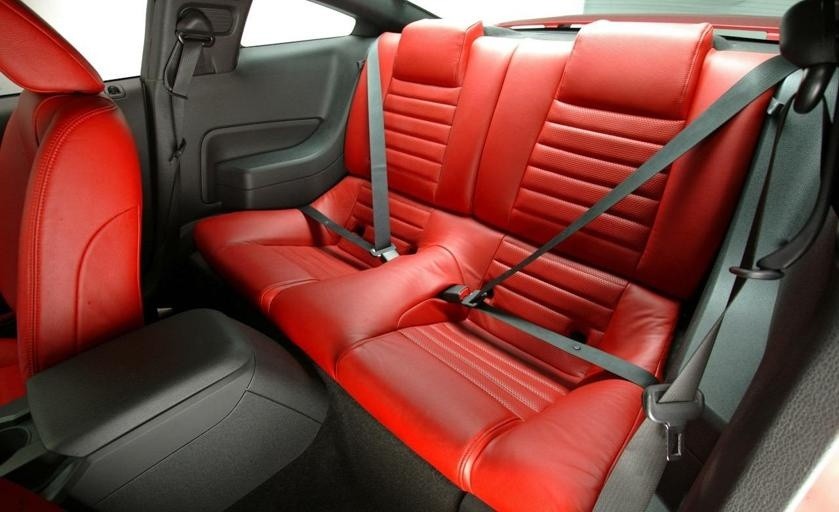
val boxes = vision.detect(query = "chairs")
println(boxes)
[2,0,144,414]
